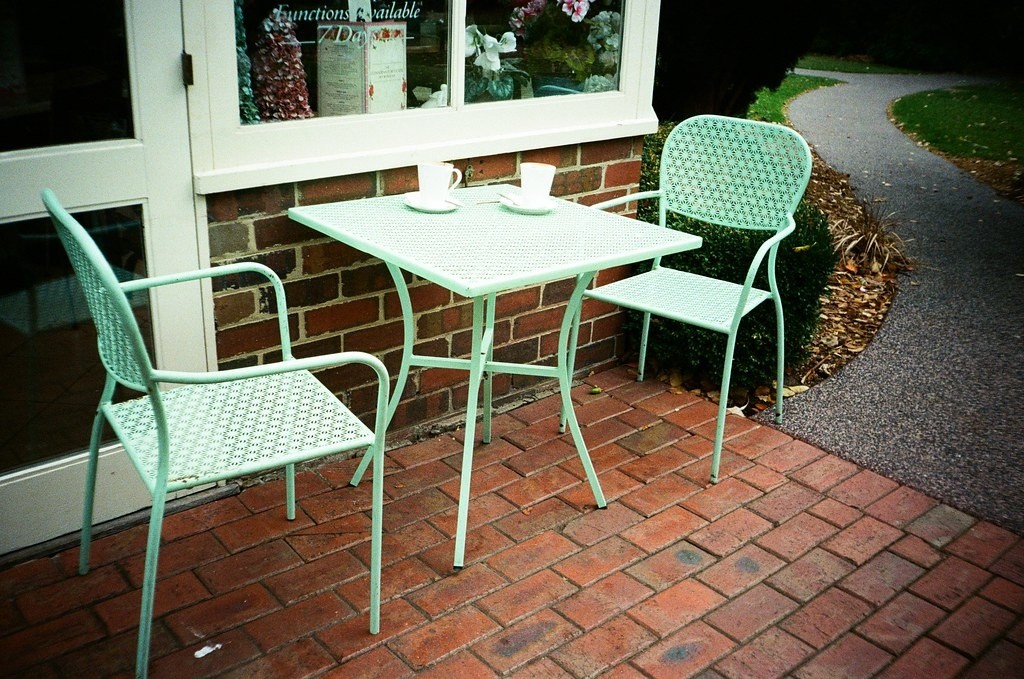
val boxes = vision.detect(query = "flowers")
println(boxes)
[508,0,623,69]
[465,25,530,77]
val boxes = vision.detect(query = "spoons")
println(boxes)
[494,190,520,206]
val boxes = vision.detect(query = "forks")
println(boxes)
[444,198,500,207]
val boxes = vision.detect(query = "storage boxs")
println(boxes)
[316,19,408,116]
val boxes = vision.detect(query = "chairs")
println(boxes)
[555,113,813,486]
[40,187,390,679]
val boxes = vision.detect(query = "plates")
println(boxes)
[501,196,559,215]
[403,191,460,214]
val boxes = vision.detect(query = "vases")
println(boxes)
[524,42,596,90]
[464,74,514,103]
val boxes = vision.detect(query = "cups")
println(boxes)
[520,162,556,206]
[417,163,462,206]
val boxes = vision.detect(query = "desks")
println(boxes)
[287,184,703,571]
